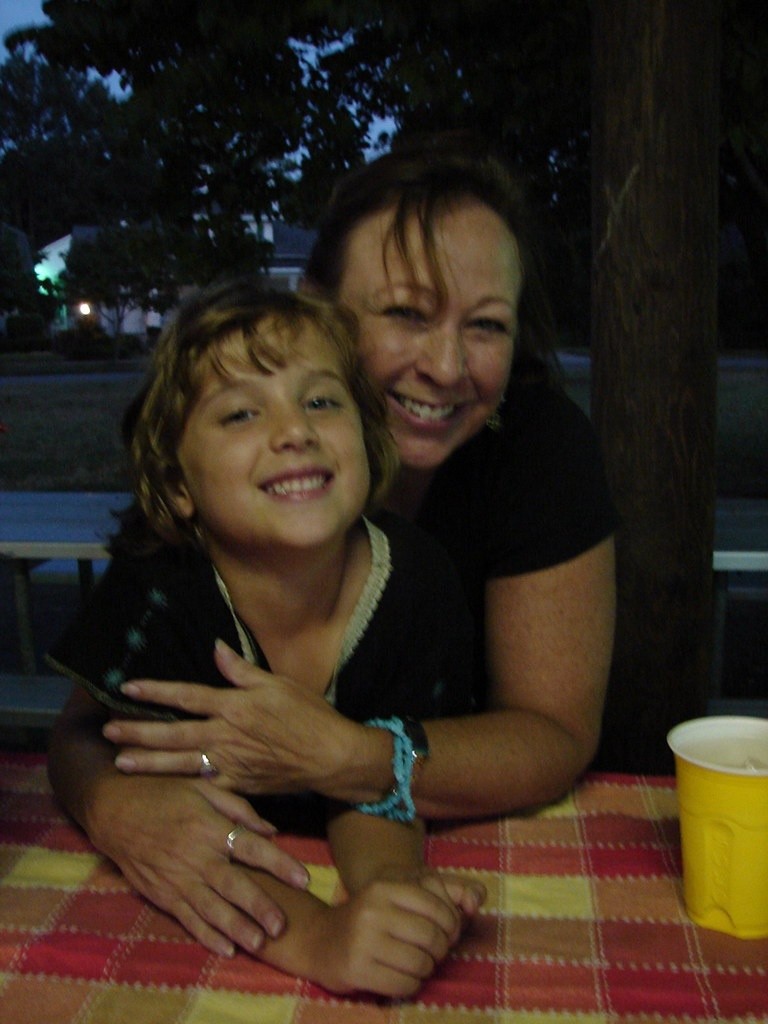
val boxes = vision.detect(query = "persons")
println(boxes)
[41,132,620,955]
[101,286,484,1000]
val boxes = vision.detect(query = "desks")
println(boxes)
[0,493,768,675]
[0,751,768,1024]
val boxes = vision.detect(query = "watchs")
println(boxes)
[383,711,429,806]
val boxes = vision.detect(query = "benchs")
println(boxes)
[0,676,78,729]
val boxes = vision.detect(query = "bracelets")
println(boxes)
[355,711,415,825]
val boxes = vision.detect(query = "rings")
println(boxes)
[202,754,211,766]
[226,824,247,855]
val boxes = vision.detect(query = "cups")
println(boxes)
[666,714,768,941]
